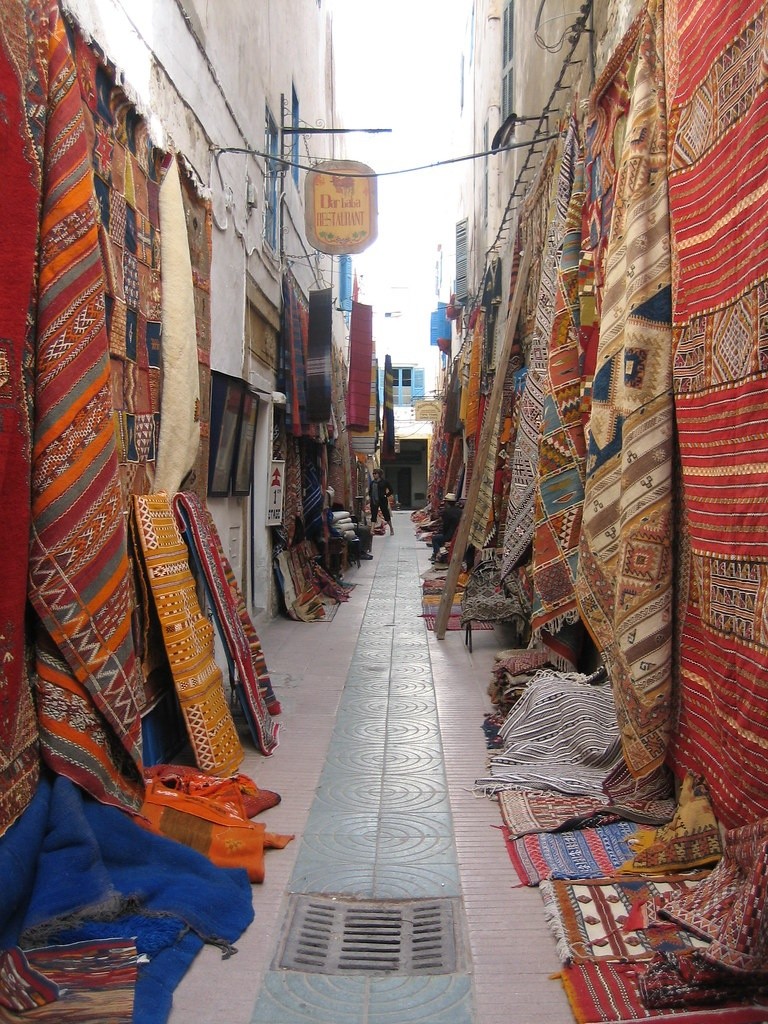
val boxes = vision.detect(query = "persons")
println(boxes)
[369,468,394,535]
[430,493,462,560]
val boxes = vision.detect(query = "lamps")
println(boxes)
[492,114,549,157]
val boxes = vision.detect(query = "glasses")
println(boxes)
[373,472,378,475]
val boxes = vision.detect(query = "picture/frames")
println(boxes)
[208,384,244,497]
[231,388,260,498]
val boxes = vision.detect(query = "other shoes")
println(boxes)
[428,556,436,561]
[390,527,394,535]
[370,531,375,535]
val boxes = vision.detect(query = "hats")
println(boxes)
[442,493,456,501]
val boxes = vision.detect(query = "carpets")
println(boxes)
[411,504,503,631]
[486,656,768,1024]
[1,772,293,1023]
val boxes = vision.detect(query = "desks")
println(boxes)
[466,615,523,653]
